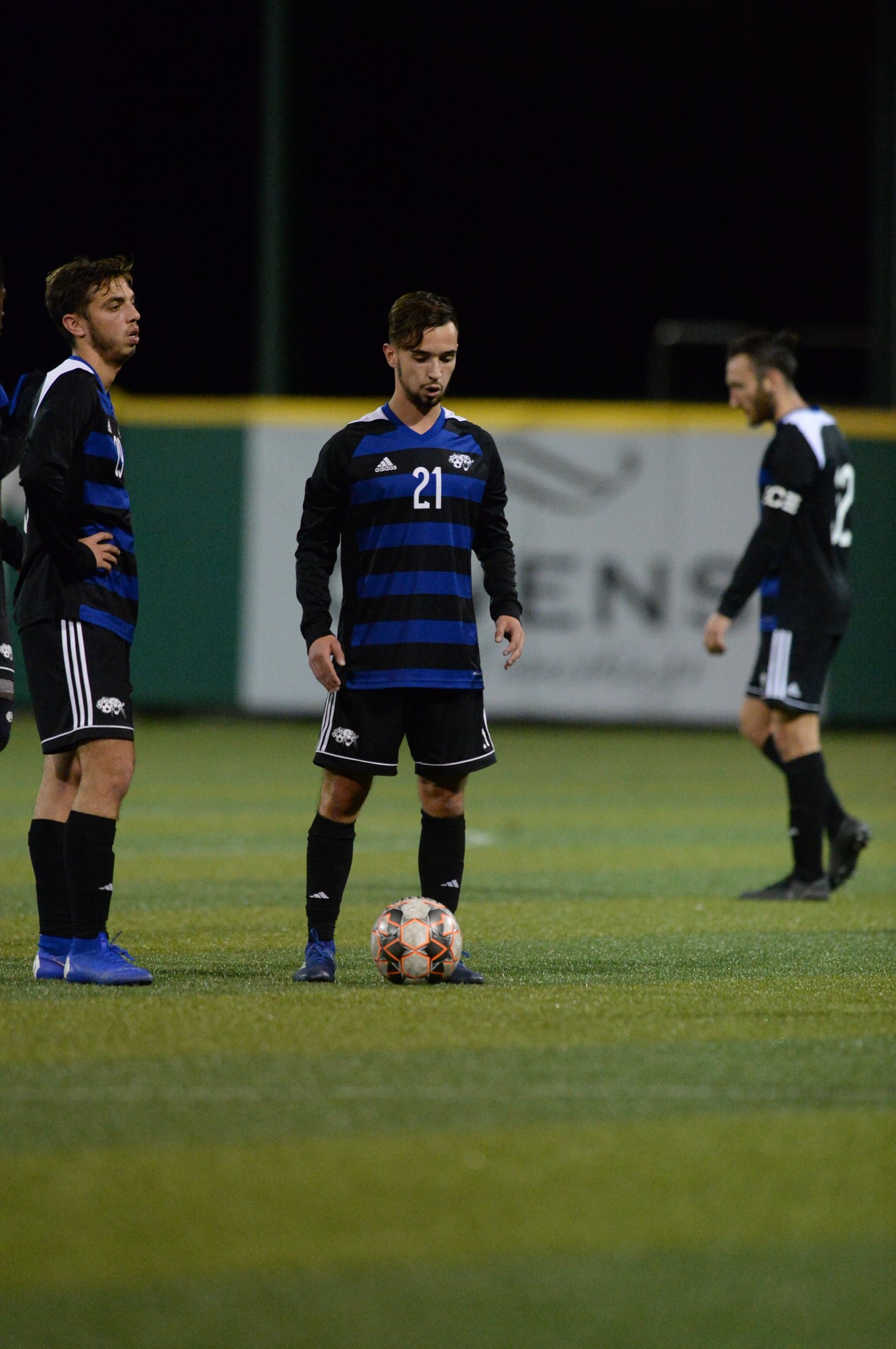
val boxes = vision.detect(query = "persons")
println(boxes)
[702,332,872,902]
[1,257,154,985]
[293,291,526,986]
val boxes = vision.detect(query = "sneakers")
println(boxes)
[741,870,828,902]
[33,932,69,980]
[62,930,152,985]
[447,951,483,983]
[829,818,870,889]
[291,925,336,982]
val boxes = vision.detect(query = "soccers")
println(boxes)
[369,895,462,985]
